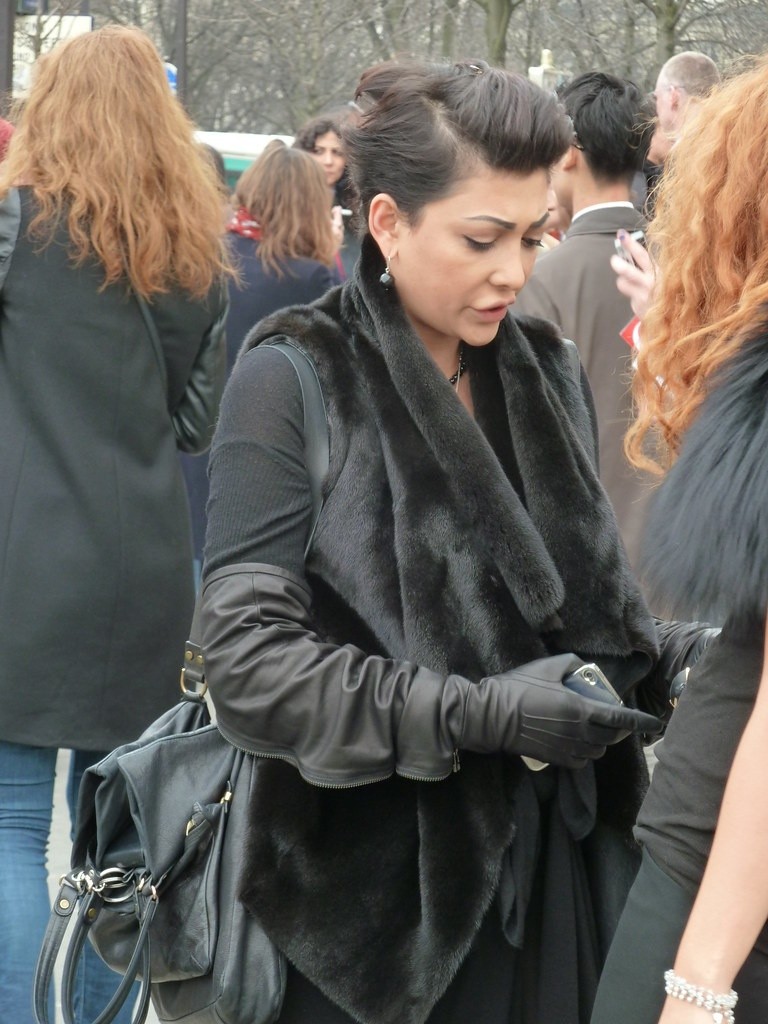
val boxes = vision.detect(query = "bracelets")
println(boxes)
[661,968,739,1024]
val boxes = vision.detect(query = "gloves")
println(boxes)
[670,626,721,708]
[197,561,666,788]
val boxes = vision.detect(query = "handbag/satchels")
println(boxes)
[32,336,330,1024]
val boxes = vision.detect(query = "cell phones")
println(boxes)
[521,663,625,771]
[615,230,649,271]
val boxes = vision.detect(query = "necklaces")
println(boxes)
[447,346,467,393]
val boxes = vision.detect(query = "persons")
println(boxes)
[178,138,340,567]
[657,51,722,132]
[1,22,237,1024]
[586,54,768,1024]
[199,62,728,1024]
[508,70,678,580]
[286,121,365,284]
[627,90,673,218]
[611,226,675,401]
